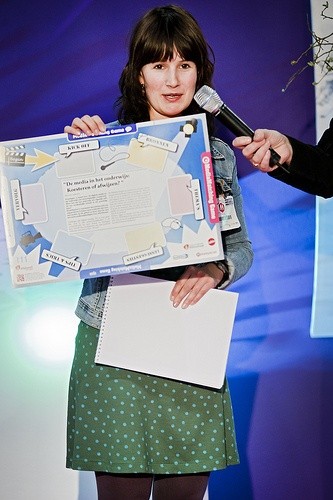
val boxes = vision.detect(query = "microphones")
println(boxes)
[195,85,281,168]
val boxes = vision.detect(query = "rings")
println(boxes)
[251,160,260,167]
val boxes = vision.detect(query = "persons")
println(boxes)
[232,112,333,199]
[56,4,257,500]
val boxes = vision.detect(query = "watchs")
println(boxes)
[205,258,228,288]
